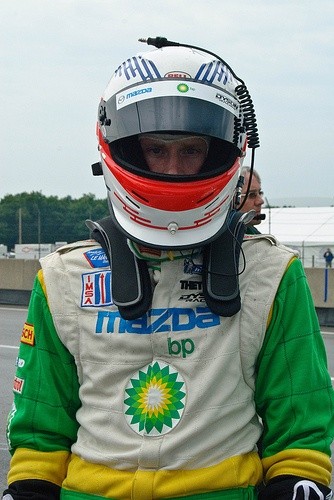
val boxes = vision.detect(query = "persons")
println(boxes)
[236,166,264,226]
[323,248,334,269]
[2,38,334,500]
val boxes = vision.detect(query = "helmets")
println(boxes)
[92,36,261,247]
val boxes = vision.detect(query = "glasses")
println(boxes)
[241,191,264,200]
